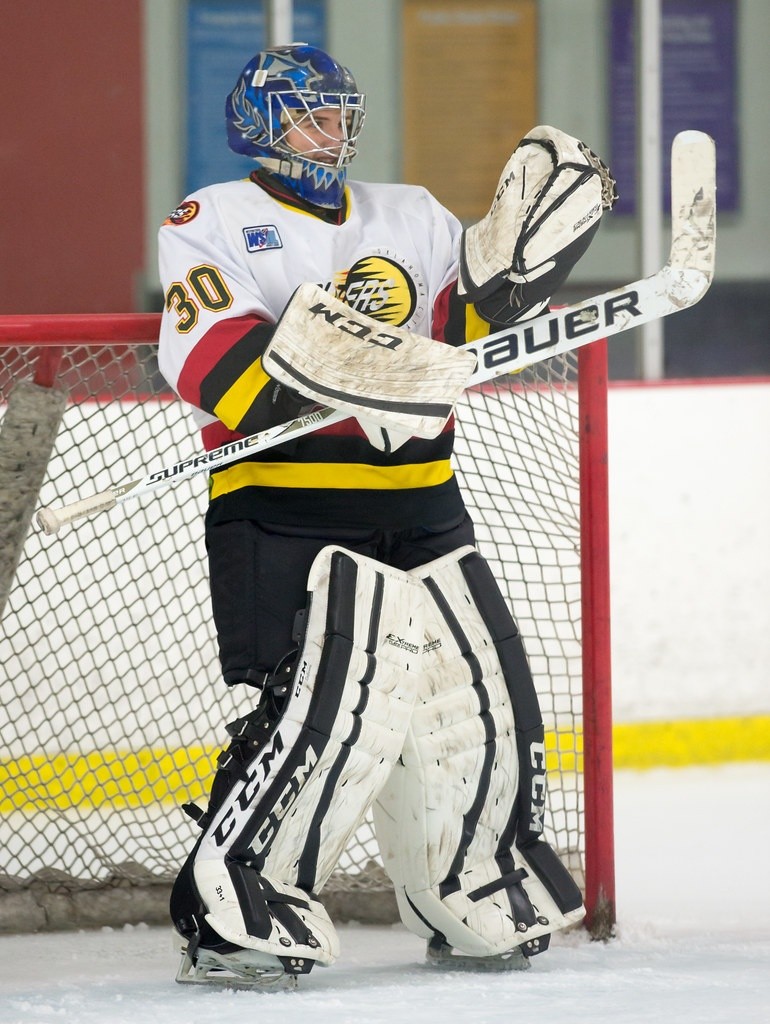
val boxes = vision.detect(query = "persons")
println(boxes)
[152,41,616,991]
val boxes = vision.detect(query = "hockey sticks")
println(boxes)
[34,128,720,538]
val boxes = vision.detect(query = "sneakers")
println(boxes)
[170,924,299,993]
[427,942,532,974]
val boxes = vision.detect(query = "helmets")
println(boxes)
[225,42,358,208]
